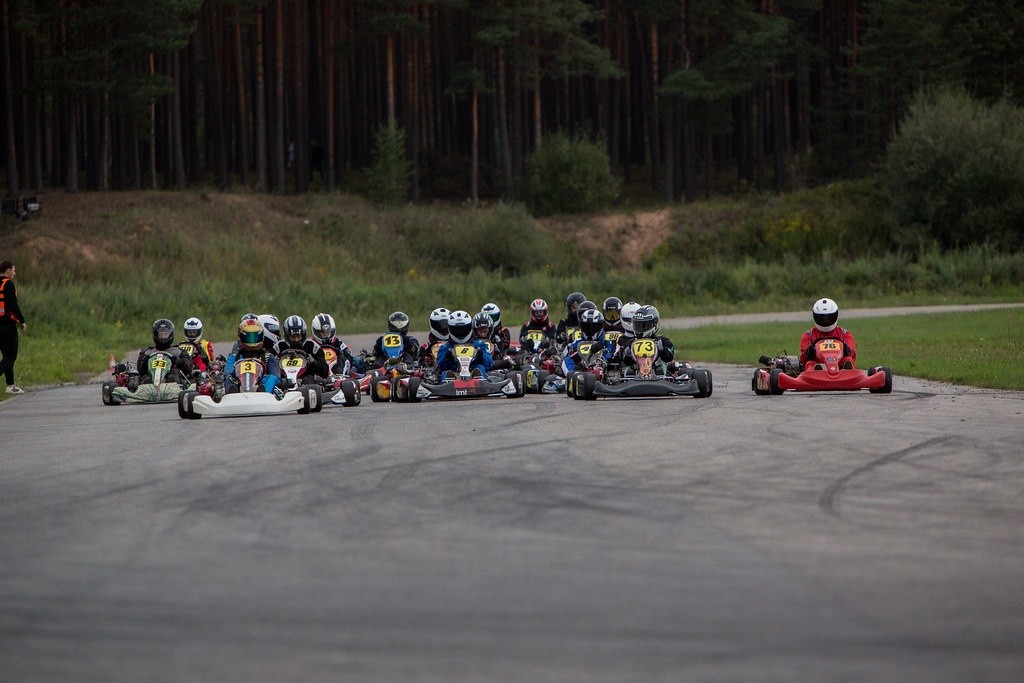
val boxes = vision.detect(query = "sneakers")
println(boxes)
[5,385,24,394]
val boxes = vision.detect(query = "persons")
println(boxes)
[224,312,353,393]
[418,303,511,383]
[519,299,556,345]
[183,318,214,371]
[373,312,419,367]
[0,260,27,393]
[555,293,674,377]
[137,319,192,384]
[800,298,856,371]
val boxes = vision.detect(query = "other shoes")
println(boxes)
[471,368,482,379]
[446,370,457,379]
[256,385,266,392]
[228,384,238,394]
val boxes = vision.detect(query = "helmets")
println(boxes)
[283,315,308,347]
[429,308,451,340]
[529,299,548,324]
[811,298,839,333]
[481,303,501,330]
[312,313,337,345]
[447,310,473,345]
[240,313,258,323]
[152,319,175,346]
[257,315,282,343]
[473,312,494,340]
[183,317,203,342]
[566,292,661,338]
[388,311,410,336]
[238,319,265,352]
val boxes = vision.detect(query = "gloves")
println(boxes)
[139,337,665,366]
[806,346,816,360]
[842,344,849,357]
